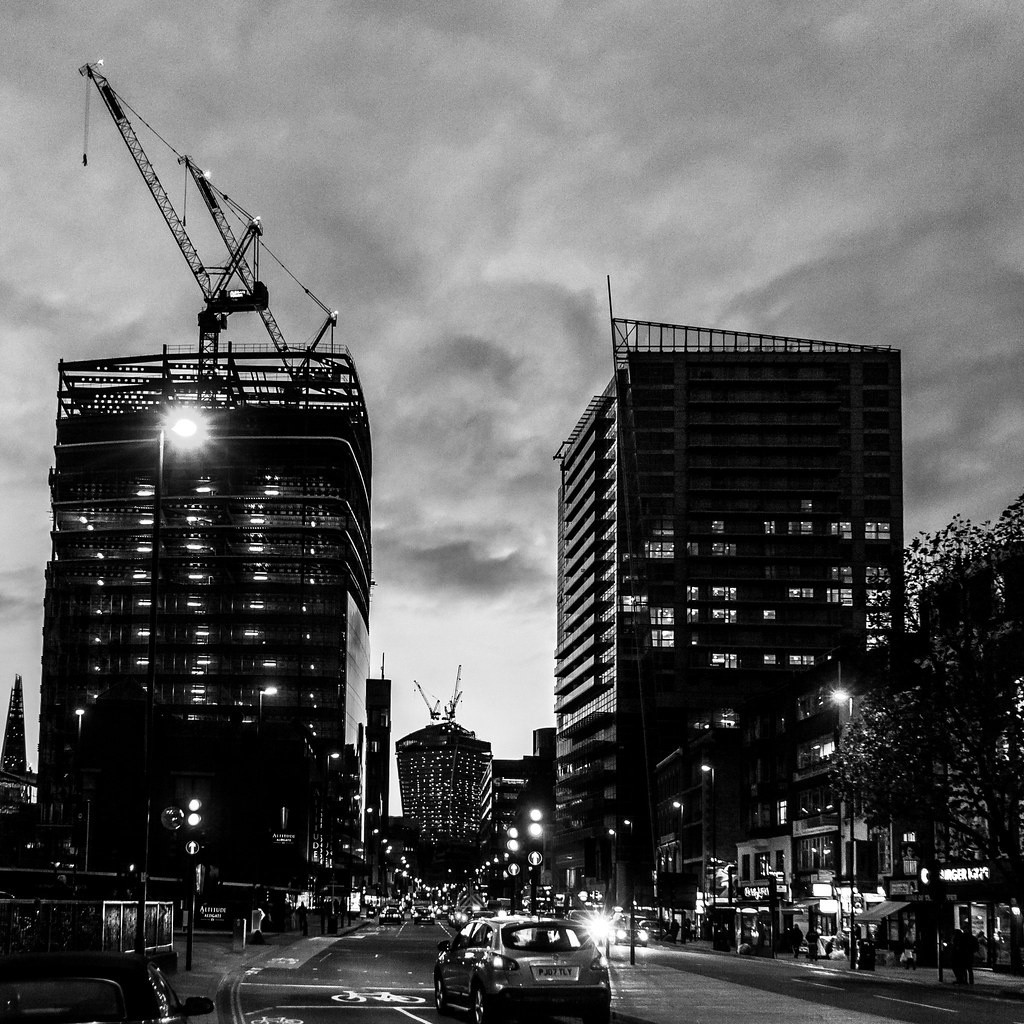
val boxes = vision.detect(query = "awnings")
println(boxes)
[855,901,912,925]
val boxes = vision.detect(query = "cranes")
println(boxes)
[77,58,338,417]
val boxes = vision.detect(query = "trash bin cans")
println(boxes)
[327,914,340,935]
[859,938,874,971]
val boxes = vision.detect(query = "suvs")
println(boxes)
[433,915,612,1024]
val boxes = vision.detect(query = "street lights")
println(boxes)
[624,819,634,965]
[74,706,86,738]
[181,797,206,970]
[327,753,341,774]
[260,684,279,720]
[832,689,854,934]
[506,828,522,917]
[702,763,715,906]
[672,800,684,873]
[608,829,617,905]
[526,806,545,916]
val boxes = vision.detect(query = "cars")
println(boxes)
[379,900,496,932]
[0,950,216,1024]
[562,909,667,947]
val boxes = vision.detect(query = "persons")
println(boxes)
[297,897,351,931]
[668,915,1003,986]
